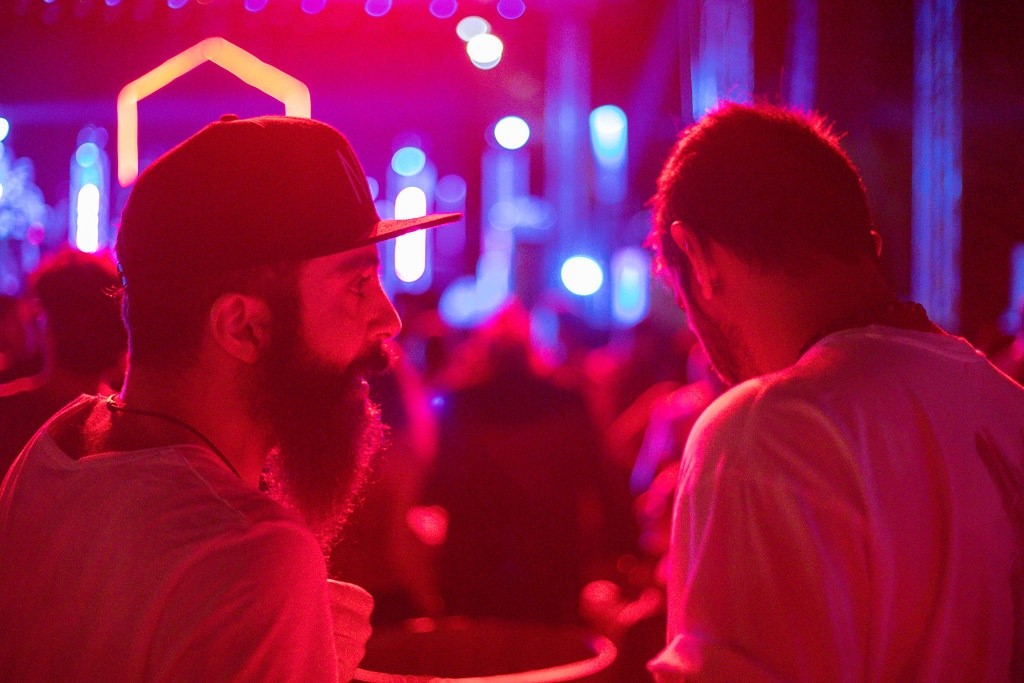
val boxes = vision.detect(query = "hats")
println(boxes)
[117,112,465,305]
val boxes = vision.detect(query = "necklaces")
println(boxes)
[106,395,242,478]
[798,298,927,359]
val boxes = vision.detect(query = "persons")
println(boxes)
[646,101,1024,683]
[0,114,462,683]
[0,249,1024,631]
